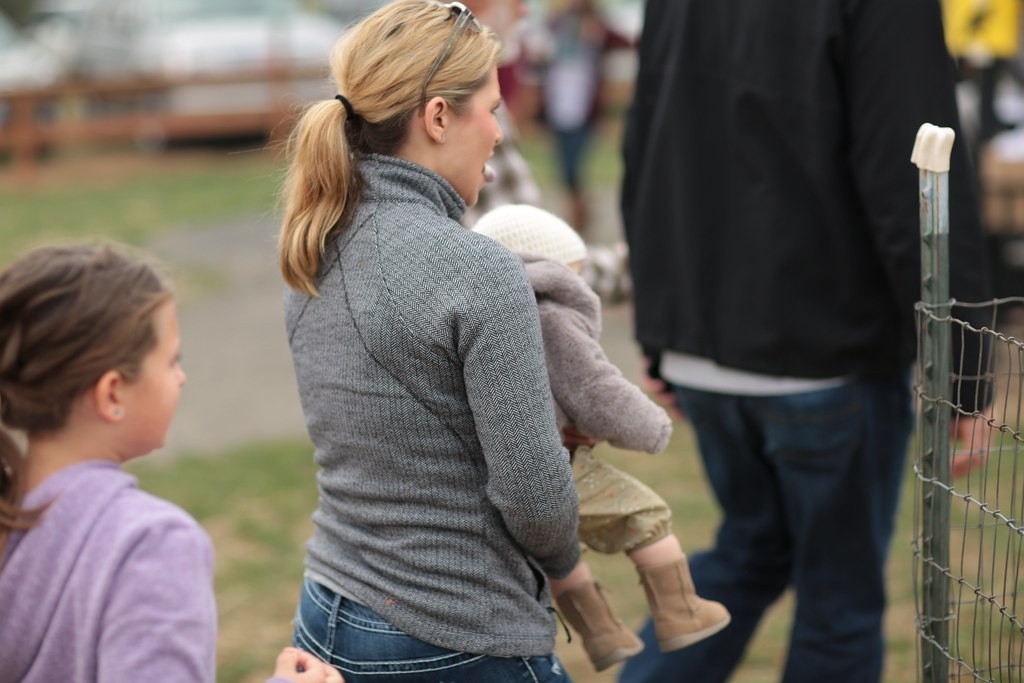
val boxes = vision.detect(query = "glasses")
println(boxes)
[417,1,484,118]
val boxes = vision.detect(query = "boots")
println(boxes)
[635,555,731,653]
[554,580,646,673]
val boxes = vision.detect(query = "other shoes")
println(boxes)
[572,197,591,235]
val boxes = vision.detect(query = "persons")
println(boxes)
[275,1,583,683]
[472,203,730,674]
[616,0,999,682]
[1,245,218,683]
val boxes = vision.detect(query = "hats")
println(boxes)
[472,203,588,264]
